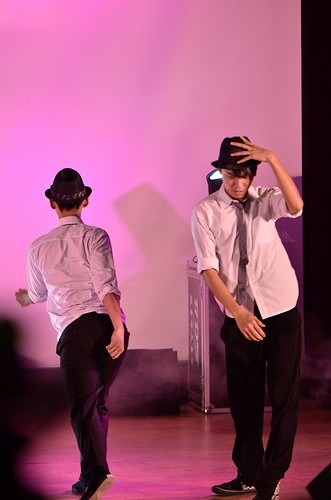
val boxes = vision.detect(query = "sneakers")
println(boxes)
[80,473,115,500]
[211,478,256,495]
[72,477,83,492]
[251,479,282,500]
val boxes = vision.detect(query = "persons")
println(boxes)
[14,167,130,500]
[191,136,304,499]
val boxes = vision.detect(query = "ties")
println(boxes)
[231,198,252,307]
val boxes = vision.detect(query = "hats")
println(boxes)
[45,168,92,204]
[210,135,262,170]
